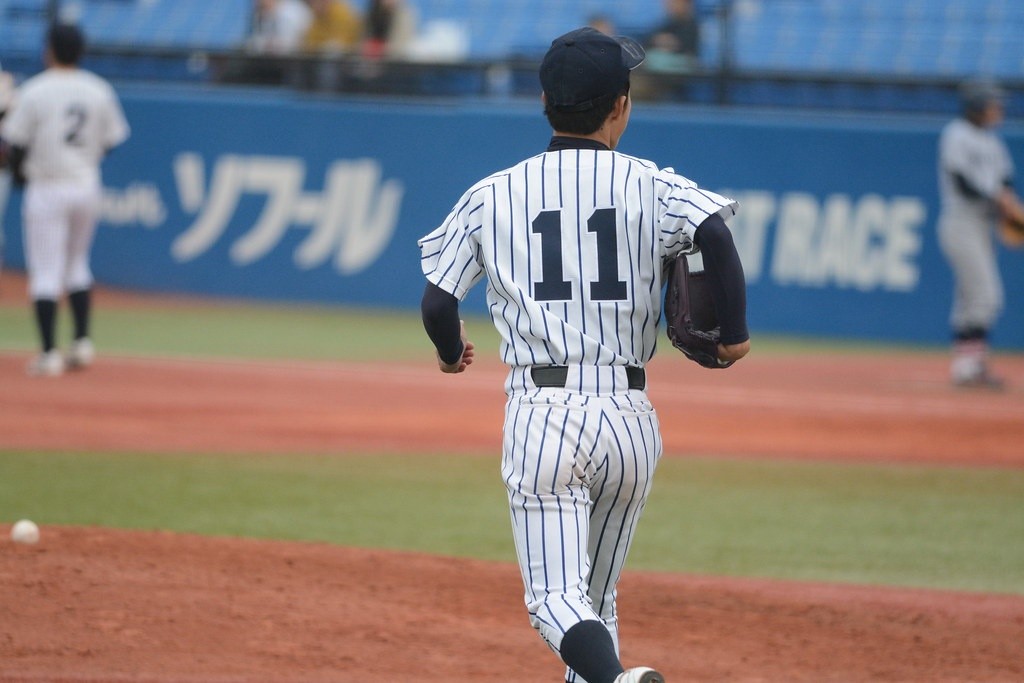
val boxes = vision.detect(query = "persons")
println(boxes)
[237,1,420,93]
[0,27,132,376]
[416,29,750,683]
[935,80,1023,386]
[589,0,723,102]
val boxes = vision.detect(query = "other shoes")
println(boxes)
[31,349,64,376]
[951,359,999,389]
[70,337,93,364]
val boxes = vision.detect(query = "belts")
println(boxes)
[533,366,645,390]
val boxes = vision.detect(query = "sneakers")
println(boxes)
[614,666,665,683]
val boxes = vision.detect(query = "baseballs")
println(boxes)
[12,518,40,544]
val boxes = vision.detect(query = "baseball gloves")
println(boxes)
[663,253,736,370]
[998,217,1024,245]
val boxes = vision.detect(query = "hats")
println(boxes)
[537,25,647,108]
